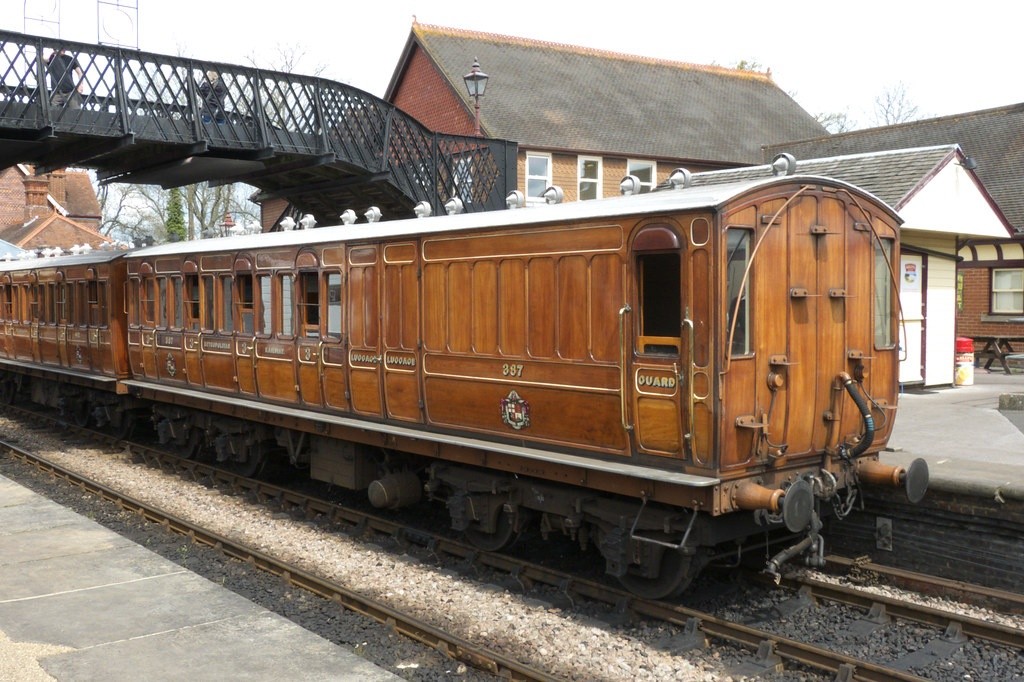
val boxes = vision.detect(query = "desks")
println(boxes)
[960,335,1024,375]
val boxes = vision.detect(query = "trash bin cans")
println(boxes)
[954,337,975,388]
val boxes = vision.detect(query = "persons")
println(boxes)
[45,49,83,110]
[201,71,226,125]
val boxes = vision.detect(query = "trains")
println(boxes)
[1,155,929,607]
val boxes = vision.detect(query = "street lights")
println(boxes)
[463,57,492,137]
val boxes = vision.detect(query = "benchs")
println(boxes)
[972,349,1024,359]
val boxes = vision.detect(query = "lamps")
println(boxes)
[954,153,977,169]
[462,56,489,136]
